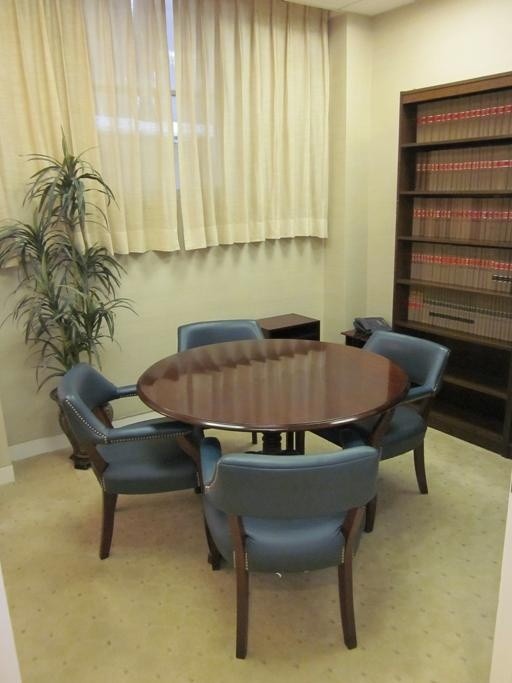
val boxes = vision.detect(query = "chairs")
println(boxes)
[293,330,451,532]
[56,362,219,571]
[196,435,383,659]
[175,316,294,452]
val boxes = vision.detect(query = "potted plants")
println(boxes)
[0,125,140,470]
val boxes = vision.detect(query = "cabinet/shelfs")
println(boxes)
[392,70,511,458]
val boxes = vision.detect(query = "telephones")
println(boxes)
[353,317,392,338]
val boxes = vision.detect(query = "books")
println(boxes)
[407,87,512,343]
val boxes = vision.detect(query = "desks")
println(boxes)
[341,326,373,347]
[256,311,321,339]
[137,336,411,455]
